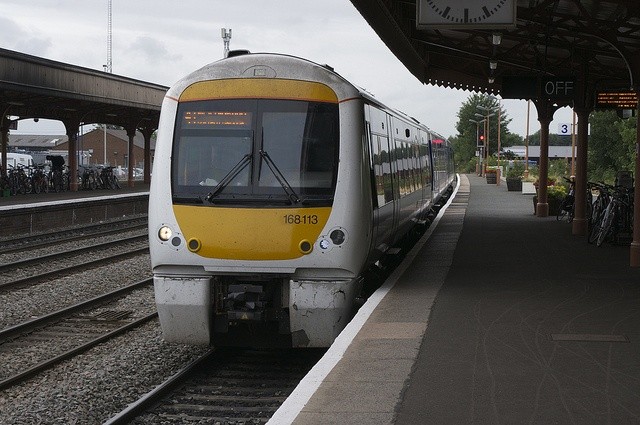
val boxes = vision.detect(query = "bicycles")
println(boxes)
[0,163,122,196]
[556,175,634,246]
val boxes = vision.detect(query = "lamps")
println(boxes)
[488,77,494,83]
[490,60,498,69]
[492,32,502,46]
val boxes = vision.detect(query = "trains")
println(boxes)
[148,49,458,350]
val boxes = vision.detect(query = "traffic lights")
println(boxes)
[479,130,484,146]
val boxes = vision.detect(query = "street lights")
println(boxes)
[474,113,496,177]
[468,118,488,175]
[475,105,501,179]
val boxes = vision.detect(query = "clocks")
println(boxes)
[416,0,516,30]
